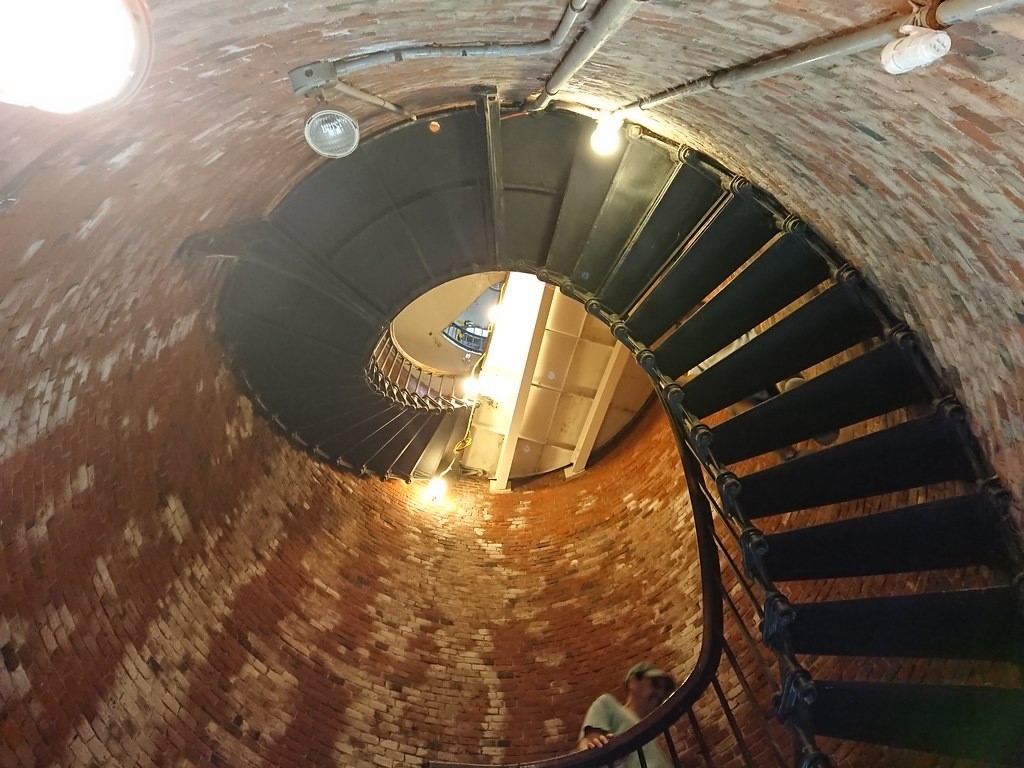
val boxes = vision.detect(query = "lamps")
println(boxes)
[287,61,360,159]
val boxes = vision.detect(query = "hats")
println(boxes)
[625,661,675,693]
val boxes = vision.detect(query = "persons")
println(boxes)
[578,661,675,768]
